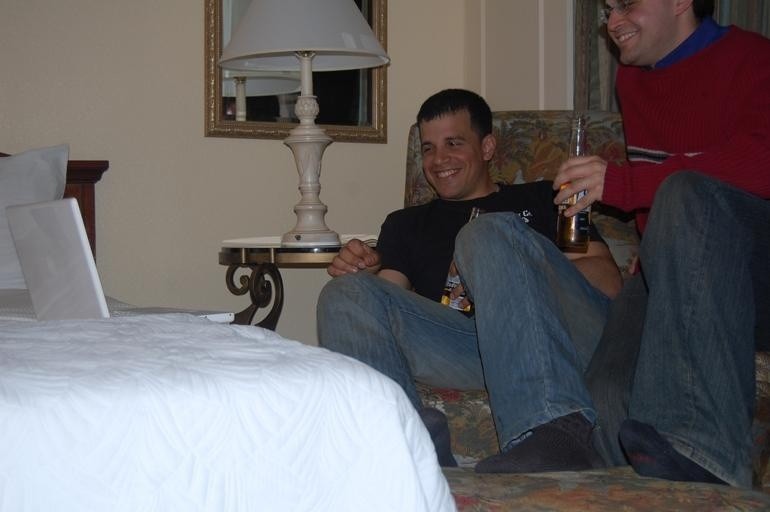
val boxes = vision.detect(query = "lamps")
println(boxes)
[218,2,390,249]
[219,5,302,121]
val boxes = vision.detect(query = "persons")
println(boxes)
[551,0,770,489]
[315,88,624,474]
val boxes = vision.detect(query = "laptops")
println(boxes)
[4,197,235,324]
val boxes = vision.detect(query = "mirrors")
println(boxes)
[203,1,388,144]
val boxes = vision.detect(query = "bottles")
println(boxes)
[555,118,591,252]
[440,206,483,317]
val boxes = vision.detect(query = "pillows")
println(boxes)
[1,142,69,292]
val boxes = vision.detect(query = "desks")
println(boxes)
[216,239,377,329]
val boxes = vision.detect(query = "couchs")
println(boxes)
[404,109,769,511]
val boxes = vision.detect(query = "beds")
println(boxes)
[0,155,455,511]
